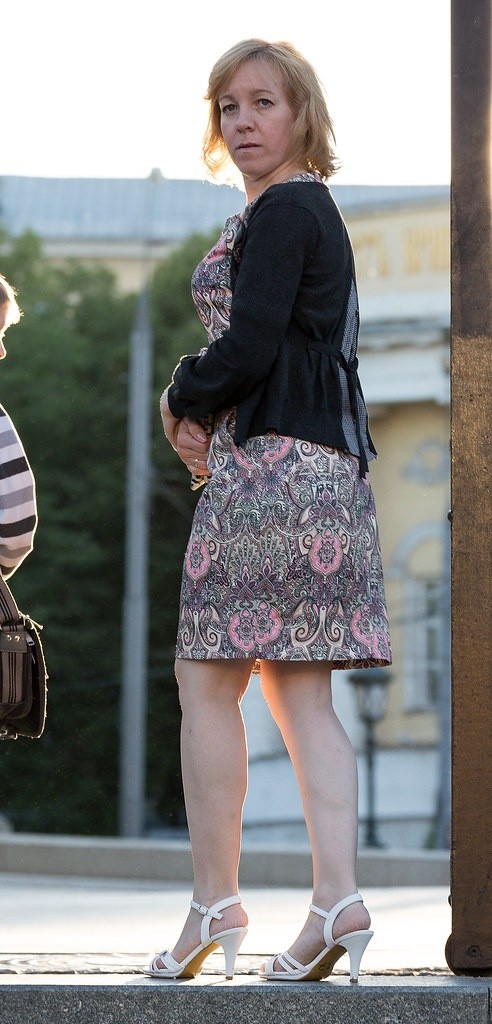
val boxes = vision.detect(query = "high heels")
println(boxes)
[259,894,374,982]
[143,895,247,978]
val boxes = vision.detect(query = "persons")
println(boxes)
[144,38,394,987]
[0,275,39,579]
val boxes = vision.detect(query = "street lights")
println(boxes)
[349,666,391,853]
[122,232,176,837]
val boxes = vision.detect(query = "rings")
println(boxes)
[195,459,198,467]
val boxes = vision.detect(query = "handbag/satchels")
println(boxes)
[0,576,47,740]
